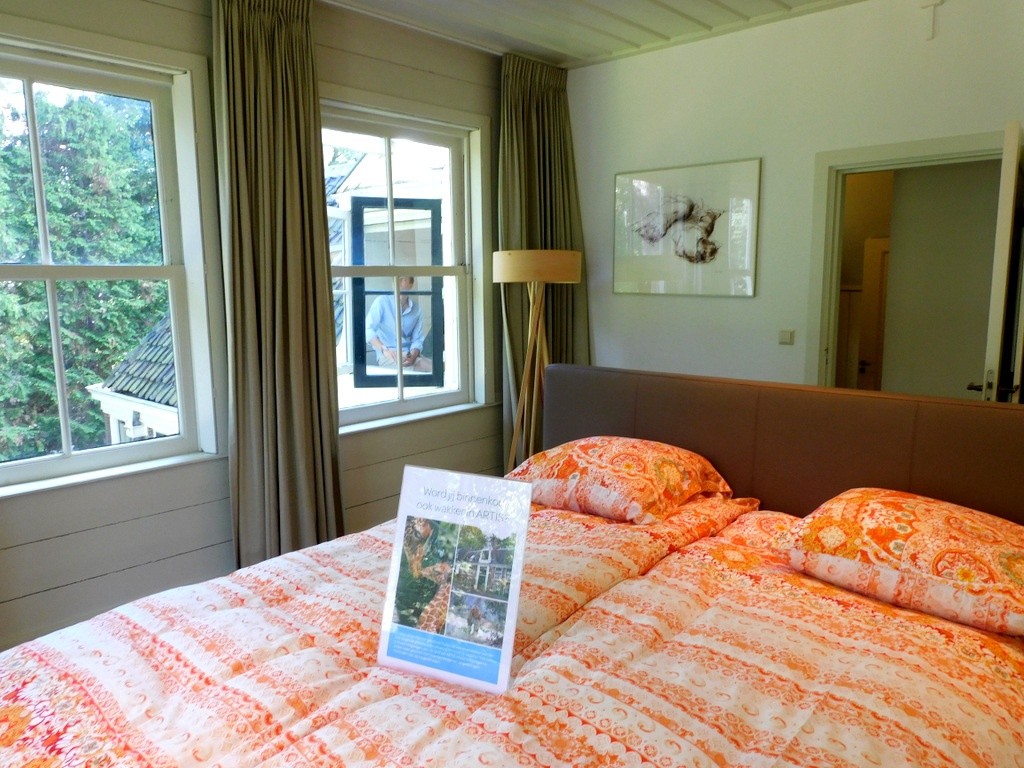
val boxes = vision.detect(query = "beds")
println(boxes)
[0,363,1024,768]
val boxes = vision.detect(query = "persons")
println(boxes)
[365,277,432,372]
[394,516,450,625]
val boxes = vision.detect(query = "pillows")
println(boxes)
[504,435,734,524]
[782,487,1024,638]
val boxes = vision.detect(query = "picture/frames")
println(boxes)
[609,157,763,300]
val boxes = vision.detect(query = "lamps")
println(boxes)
[490,249,583,473]
